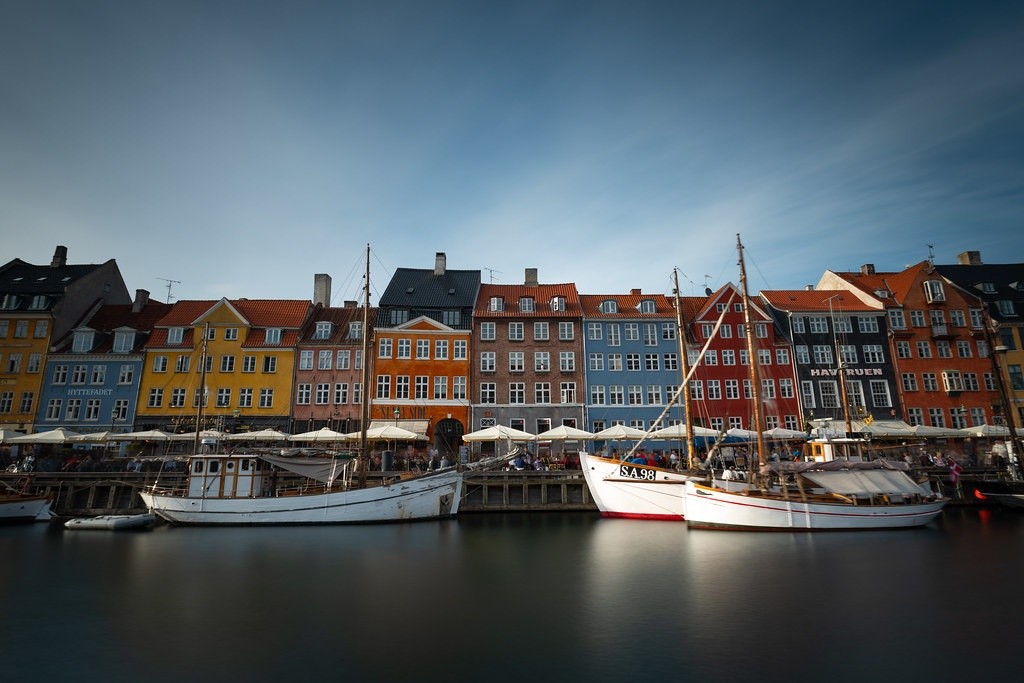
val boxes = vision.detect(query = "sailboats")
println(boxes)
[678,230,952,529]
[792,294,1023,506]
[139,247,464,527]
[579,265,789,522]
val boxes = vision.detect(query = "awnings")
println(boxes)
[913,424,1024,438]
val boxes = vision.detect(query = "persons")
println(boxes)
[561,453,574,464]
[919,453,930,466]
[510,455,549,470]
[903,451,914,464]
[993,440,1007,467]
[738,450,749,462]
[612,449,661,463]
[669,451,677,467]
[772,450,780,464]
[63,454,92,472]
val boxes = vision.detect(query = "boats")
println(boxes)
[63,505,158,532]
[0,456,55,523]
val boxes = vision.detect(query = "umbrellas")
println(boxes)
[226,428,292,450]
[289,427,356,448]
[654,423,726,458]
[536,424,594,456]
[169,430,230,455]
[461,423,536,460]
[728,426,806,457]
[348,425,429,450]
[0,427,173,460]
[595,423,653,454]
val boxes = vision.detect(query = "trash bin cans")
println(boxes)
[985,451,998,466]
[381,450,392,471]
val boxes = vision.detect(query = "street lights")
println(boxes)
[392,406,401,453]
[230,408,241,449]
[106,407,119,459]
